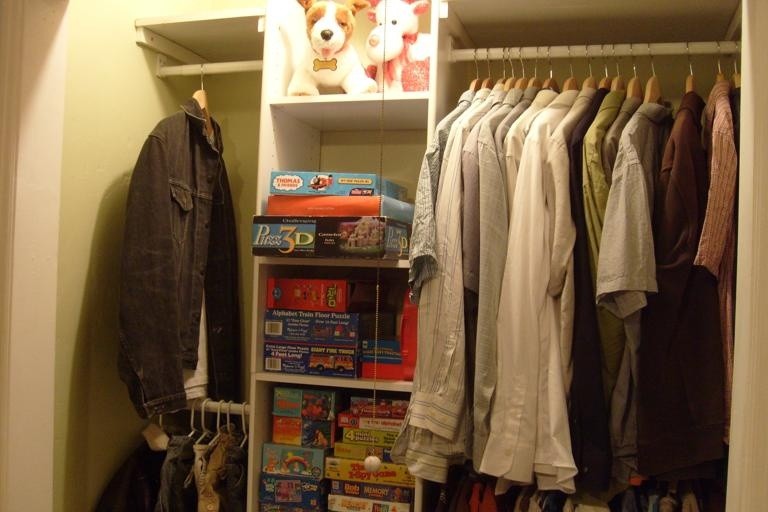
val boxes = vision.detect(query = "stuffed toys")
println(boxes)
[284,0,375,98]
[363,0,431,94]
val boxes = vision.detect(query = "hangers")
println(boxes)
[466,44,736,103]
[146,396,249,467]
[180,60,219,148]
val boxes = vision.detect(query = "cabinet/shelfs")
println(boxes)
[137,4,764,511]
